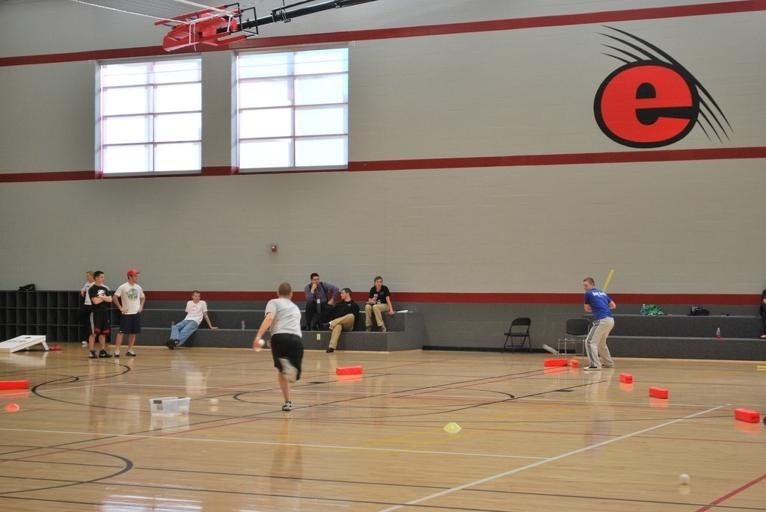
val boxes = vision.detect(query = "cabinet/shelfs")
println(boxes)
[0,289,112,343]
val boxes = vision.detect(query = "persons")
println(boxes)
[320,288,361,353]
[81,272,109,344]
[759,289,766,338]
[583,278,616,371]
[112,269,145,357]
[365,277,394,332]
[167,290,218,350]
[253,283,304,410]
[304,274,339,331]
[88,271,112,358]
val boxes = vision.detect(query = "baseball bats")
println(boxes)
[603,269,613,290]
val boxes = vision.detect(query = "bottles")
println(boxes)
[171,320,176,328]
[716,326,722,338]
[240,320,245,329]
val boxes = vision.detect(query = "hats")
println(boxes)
[128,269,140,276]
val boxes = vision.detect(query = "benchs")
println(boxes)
[583,313,765,361]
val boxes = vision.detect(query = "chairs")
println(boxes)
[503,316,532,353]
[557,318,592,357]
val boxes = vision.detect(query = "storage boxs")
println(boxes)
[148,396,191,417]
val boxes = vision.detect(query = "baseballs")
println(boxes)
[255,339,265,352]
[679,474,690,484]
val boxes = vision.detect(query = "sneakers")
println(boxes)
[366,327,371,332]
[114,350,120,357]
[601,361,614,368]
[381,324,386,332]
[327,348,334,353]
[584,365,600,370]
[167,339,179,349]
[127,351,136,356]
[282,401,294,410]
[99,350,112,357]
[89,350,97,358]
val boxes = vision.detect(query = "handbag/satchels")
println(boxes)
[689,306,709,316]
[639,303,664,316]
